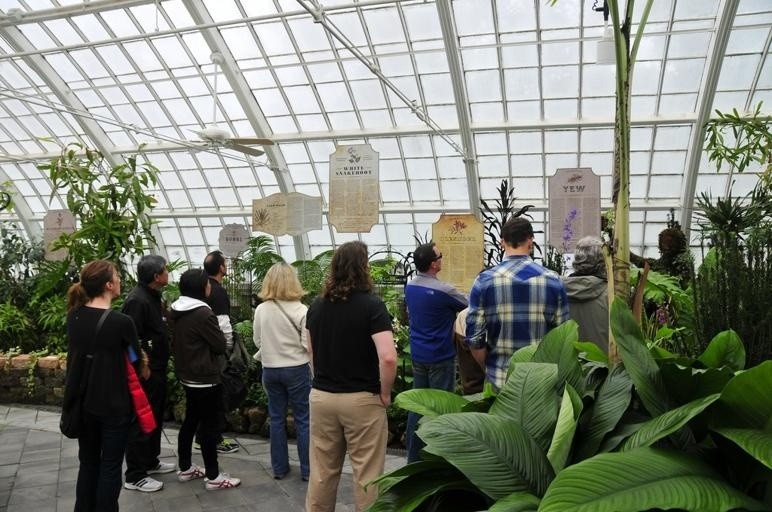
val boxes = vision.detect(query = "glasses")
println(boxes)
[434,252,442,262]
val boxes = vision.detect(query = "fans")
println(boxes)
[186,52,275,156]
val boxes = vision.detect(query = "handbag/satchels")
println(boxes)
[59,367,93,438]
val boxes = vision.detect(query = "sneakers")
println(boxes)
[176,462,206,482]
[147,461,177,475]
[204,473,241,490]
[274,470,286,479]
[216,441,240,453]
[302,472,310,480]
[194,441,202,449]
[124,475,163,492]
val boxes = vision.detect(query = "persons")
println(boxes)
[193,251,240,456]
[168,266,241,491]
[465,216,570,396]
[302,239,398,512]
[559,234,610,356]
[58,259,142,512]
[251,261,309,481]
[403,241,470,464]
[120,254,176,493]
